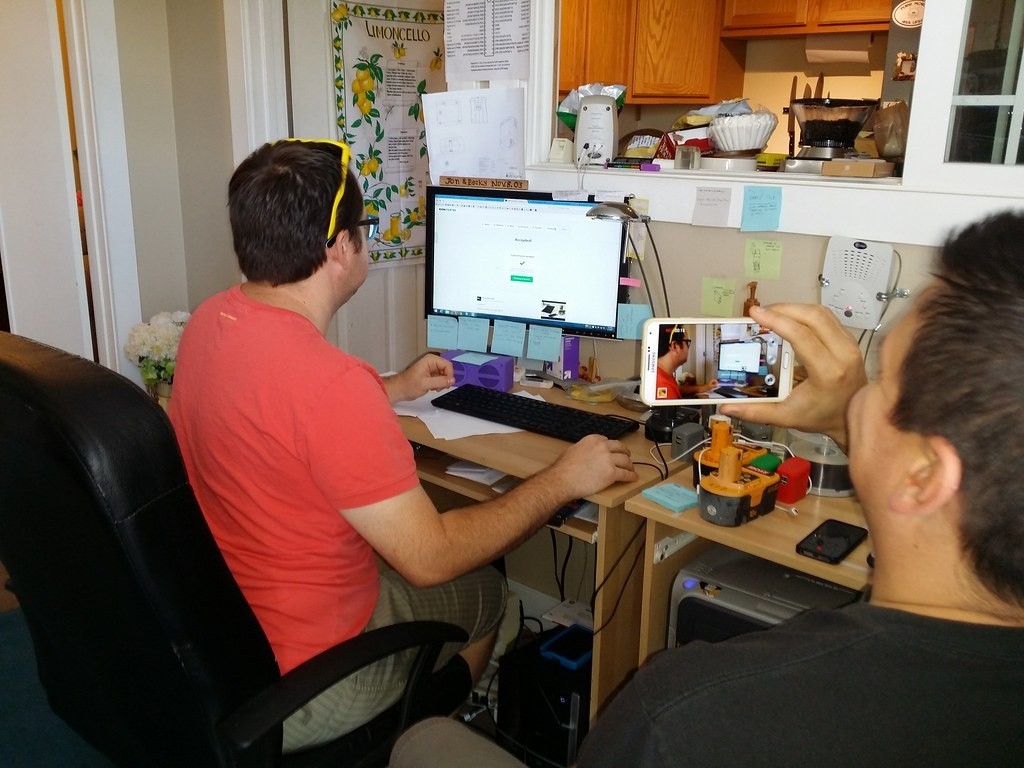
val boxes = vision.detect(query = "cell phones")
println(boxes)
[639,317,794,406]
[538,623,594,671]
[796,518,869,565]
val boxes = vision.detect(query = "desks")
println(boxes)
[625,466,875,668]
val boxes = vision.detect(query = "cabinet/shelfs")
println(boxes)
[722,1,896,39]
[398,379,692,731]
[557,0,744,108]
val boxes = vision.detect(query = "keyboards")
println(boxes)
[714,386,750,398]
[430,384,640,443]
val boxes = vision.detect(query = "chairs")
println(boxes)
[1,331,470,765]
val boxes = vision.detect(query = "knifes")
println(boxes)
[788,71,828,157]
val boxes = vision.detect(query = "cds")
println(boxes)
[786,440,851,466]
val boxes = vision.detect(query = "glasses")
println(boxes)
[326,214,378,247]
[669,339,691,348]
[269,138,353,240]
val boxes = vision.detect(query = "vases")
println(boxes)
[156,380,172,397]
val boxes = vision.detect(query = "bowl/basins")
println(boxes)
[757,153,787,172]
[791,98,878,146]
[710,113,779,152]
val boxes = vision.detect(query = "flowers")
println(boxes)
[130,311,190,390]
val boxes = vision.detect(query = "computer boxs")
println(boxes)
[495,624,592,768]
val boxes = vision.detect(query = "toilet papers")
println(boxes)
[805,31,878,64]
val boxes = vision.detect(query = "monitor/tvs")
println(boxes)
[425,185,627,339]
[717,342,762,373]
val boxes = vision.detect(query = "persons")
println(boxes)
[656,325,718,399]
[576,210,1023,767]
[170,140,637,751]
